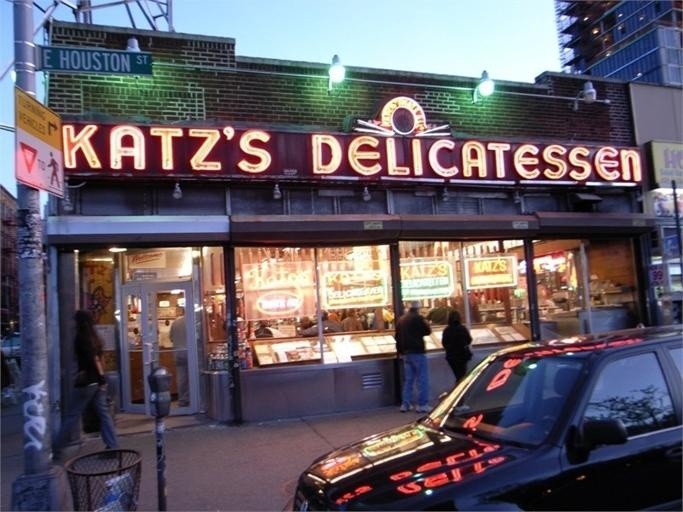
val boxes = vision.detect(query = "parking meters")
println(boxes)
[148,366,173,512]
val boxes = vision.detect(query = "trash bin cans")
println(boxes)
[579,307,632,333]
[64,448,144,512]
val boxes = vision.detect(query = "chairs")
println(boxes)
[524,366,581,424]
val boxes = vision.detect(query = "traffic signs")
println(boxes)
[32,44,155,78]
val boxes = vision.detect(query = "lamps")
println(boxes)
[571,81,599,111]
[273,184,282,200]
[362,187,371,202]
[172,183,184,201]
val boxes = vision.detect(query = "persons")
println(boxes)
[392,300,434,413]
[0,349,19,407]
[439,310,474,385]
[167,308,195,406]
[204,295,482,341]
[533,283,560,315]
[56,309,124,461]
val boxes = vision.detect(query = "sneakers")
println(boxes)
[400,404,434,413]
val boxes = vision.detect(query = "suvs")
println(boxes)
[292,324,683,511]
